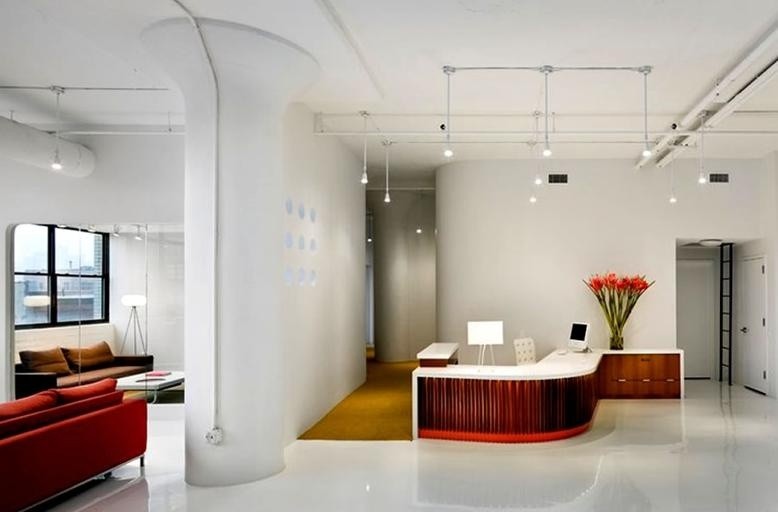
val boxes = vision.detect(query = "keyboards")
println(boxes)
[557,348,568,355]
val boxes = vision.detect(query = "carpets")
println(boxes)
[123,382,185,405]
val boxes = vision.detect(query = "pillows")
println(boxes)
[58,341,116,372]
[17,344,75,378]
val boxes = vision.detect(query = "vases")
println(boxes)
[607,336,626,351]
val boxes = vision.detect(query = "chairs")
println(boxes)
[511,336,538,368]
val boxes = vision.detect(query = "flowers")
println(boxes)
[580,266,657,349]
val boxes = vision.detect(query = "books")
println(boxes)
[145,371,171,376]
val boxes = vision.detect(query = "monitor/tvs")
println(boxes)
[568,322,590,352]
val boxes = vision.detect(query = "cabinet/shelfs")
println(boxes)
[599,351,682,399]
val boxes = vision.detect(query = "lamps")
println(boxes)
[353,108,396,208]
[117,295,151,357]
[38,85,77,173]
[434,62,658,161]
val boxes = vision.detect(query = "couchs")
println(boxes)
[0,376,152,511]
[11,341,155,400]
